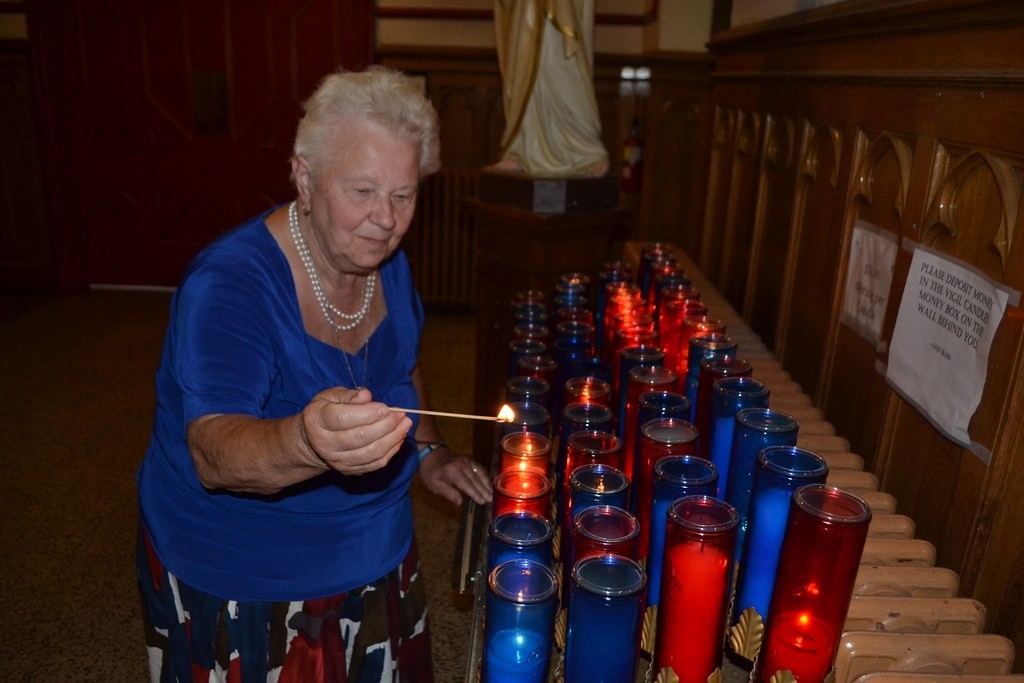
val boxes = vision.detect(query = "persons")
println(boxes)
[137,65,490,683]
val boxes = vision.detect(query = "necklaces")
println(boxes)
[286,199,378,390]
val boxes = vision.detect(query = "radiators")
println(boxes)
[623,240,1023,683]
[396,166,480,310]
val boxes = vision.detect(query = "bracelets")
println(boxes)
[418,439,445,460]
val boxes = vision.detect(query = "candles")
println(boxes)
[712,416,736,500]
[486,629,547,683]
[764,609,835,683]
[739,485,793,620]
[656,542,727,683]
